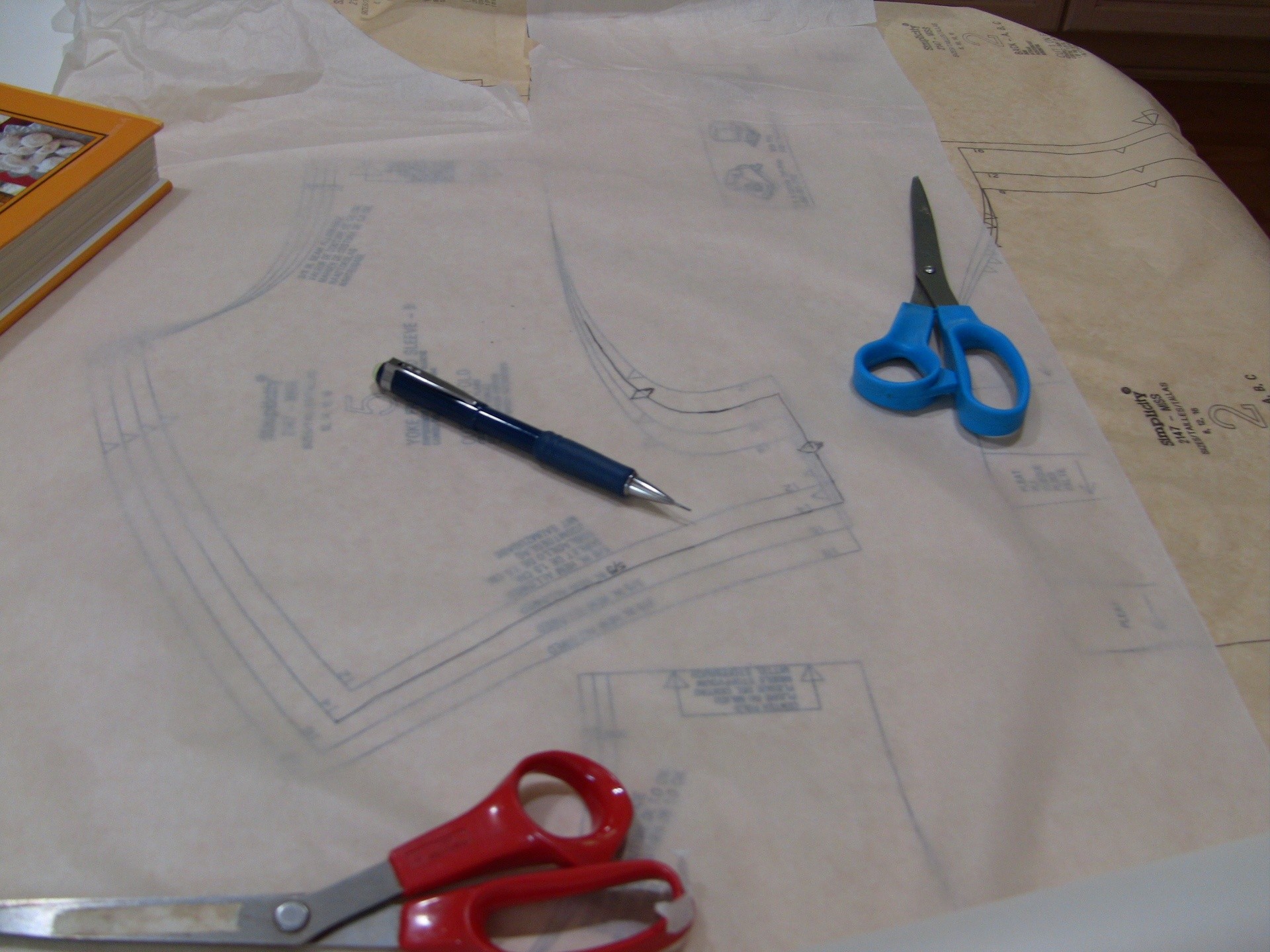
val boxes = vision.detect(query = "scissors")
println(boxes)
[0,749,695,952]
[851,175,1032,436]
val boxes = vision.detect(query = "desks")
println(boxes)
[2,0,1269,952]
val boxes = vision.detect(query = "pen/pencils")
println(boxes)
[373,357,693,513]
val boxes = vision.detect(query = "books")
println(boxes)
[0,80,170,334]
